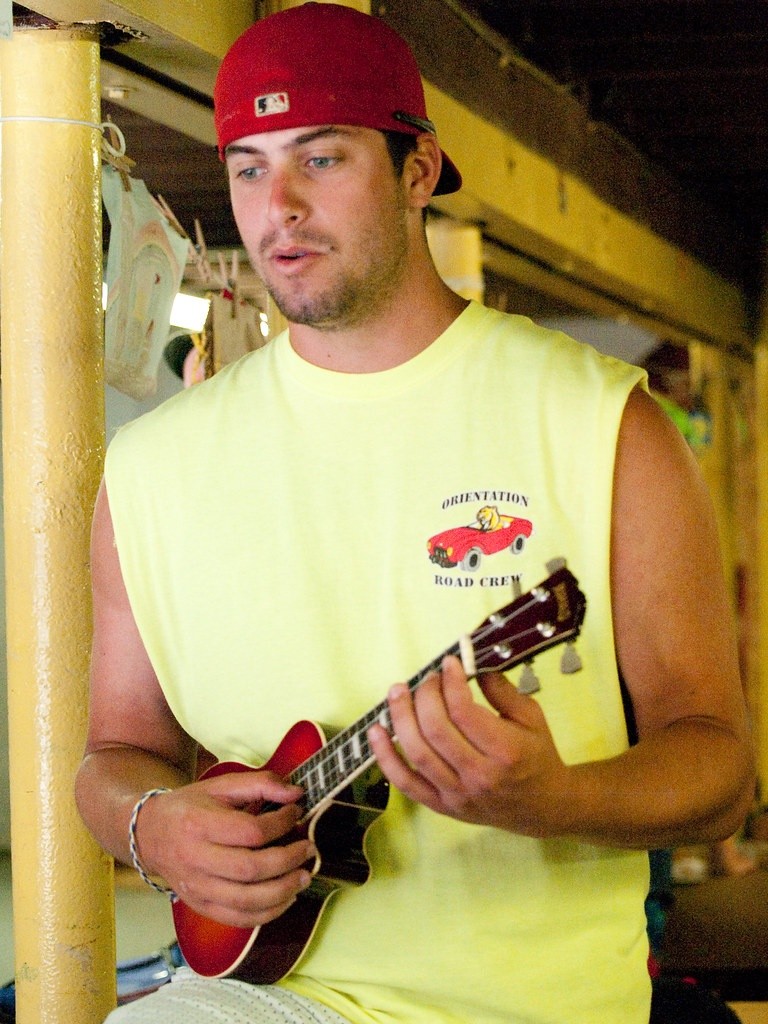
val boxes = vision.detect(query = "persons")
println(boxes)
[72,1,756,1024]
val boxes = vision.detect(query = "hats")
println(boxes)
[211,1,463,196]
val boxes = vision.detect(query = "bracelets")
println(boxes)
[126,786,180,904]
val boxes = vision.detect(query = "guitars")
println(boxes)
[169,555,589,988]
